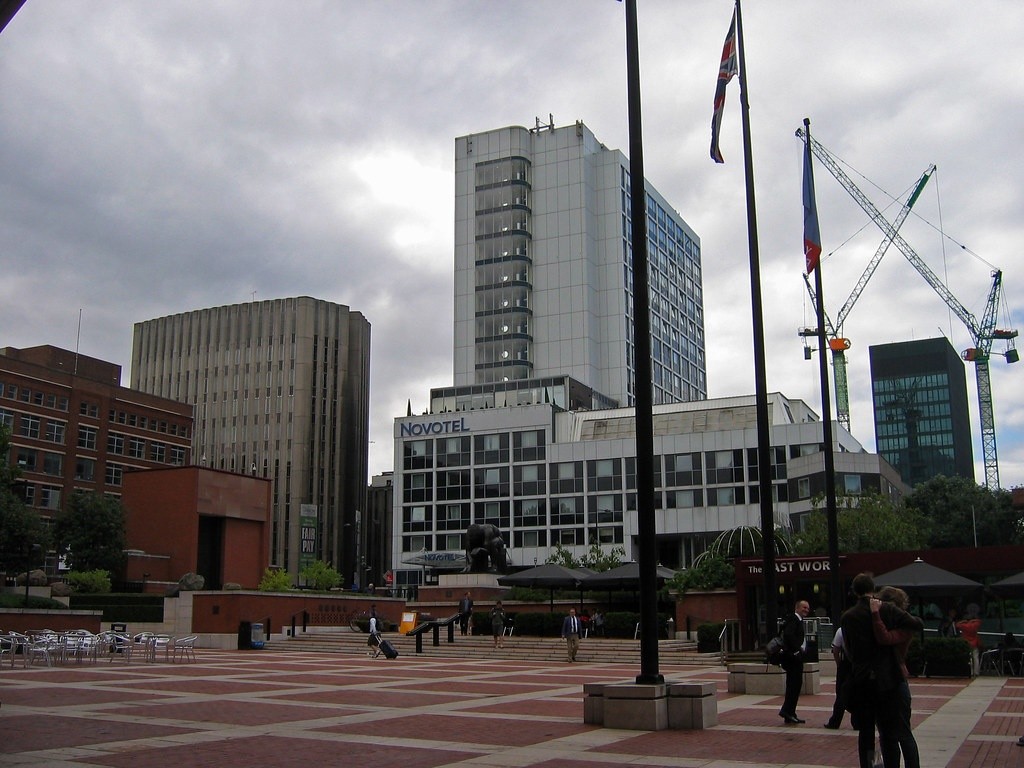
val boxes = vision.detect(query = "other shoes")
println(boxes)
[572,657,576,661]
[372,650,380,658]
[824,722,840,729]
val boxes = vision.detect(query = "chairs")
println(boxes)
[979,645,1024,677]
[0,629,199,669]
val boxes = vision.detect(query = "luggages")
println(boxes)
[374,633,399,659]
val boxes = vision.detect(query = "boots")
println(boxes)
[494,638,498,649]
[500,637,505,648]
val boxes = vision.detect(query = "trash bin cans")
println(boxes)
[110,624,126,652]
[238,622,251,649]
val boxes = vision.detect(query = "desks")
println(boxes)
[145,634,175,665]
[105,631,132,660]
[0,635,27,668]
[62,634,94,664]
[32,631,61,665]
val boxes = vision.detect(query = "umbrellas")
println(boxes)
[497,559,687,613]
[990,572,1024,587]
[871,557,983,675]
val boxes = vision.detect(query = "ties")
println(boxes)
[572,616,576,632]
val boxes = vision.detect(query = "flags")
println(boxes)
[802,117,823,276]
[710,0,746,164]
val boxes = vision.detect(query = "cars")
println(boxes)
[0,629,171,655]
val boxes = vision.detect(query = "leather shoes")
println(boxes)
[783,717,806,723]
[778,710,798,723]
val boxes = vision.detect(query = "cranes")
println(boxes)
[793,128,1021,493]
[798,164,938,433]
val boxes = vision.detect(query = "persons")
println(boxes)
[998,631,1022,677]
[579,607,604,638]
[824,627,859,730]
[489,601,506,648]
[781,600,810,724]
[841,573,924,768]
[367,613,381,658]
[561,608,581,663]
[938,602,981,677]
[370,585,419,602]
[459,524,507,574]
[458,591,474,636]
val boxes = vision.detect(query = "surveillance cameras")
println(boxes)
[203,457,206,461]
[252,467,257,471]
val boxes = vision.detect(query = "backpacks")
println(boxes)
[370,616,384,631]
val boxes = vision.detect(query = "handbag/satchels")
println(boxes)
[454,617,460,625]
[764,636,784,666]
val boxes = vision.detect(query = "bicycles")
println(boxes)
[350,611,370,633]
